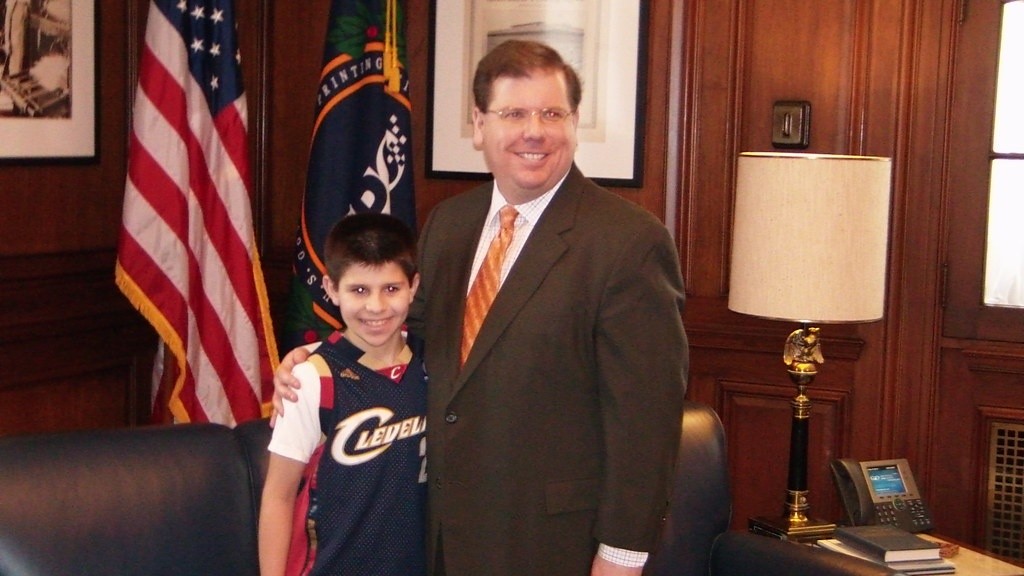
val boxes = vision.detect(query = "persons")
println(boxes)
[257,212,429,576]
[270,39,691,576]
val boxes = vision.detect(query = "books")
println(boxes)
[817,524,956,576]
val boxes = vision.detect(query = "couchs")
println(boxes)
[0,406,905,576]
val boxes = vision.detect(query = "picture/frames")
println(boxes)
[424,0,651,188]
[0,0,101,167]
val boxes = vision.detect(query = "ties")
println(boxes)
[460,205,519,369]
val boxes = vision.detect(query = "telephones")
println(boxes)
[829,456,933,533]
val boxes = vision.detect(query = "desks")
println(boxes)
[916,533,1024,576]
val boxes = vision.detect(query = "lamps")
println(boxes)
[728,151,889,542]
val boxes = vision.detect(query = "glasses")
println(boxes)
[486,107,573,124]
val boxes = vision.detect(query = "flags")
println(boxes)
[115,0,280,427]
[293,1,417,333]
